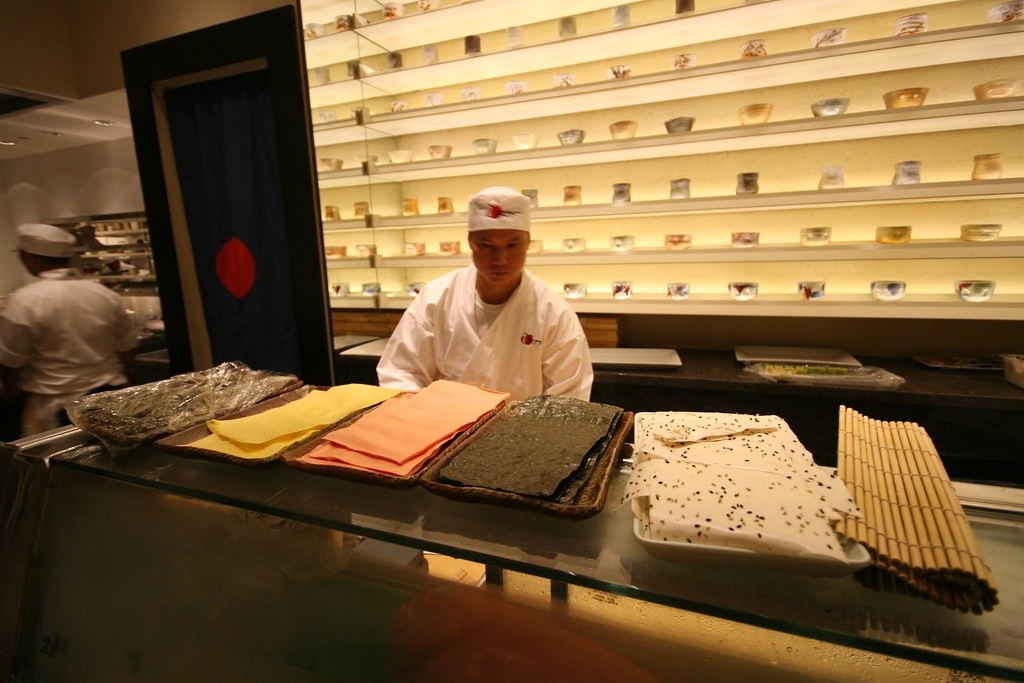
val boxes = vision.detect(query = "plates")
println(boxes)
[340,335,393,358]
[332,334,380,352]
[732,345,906,390]
[911,351,1006,372]
[590,349,682,369]
[895,13,929,35]
[988,0,1024,24]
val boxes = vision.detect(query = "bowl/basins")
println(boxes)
[959,224,1004,241]
[970,76,1022,101]
[883,87,930,111]
[870,279,906,303]
[873,226,913,245]
[954,279,996,303]
[300,0,849,303]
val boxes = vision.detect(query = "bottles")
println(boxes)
[970,153,1010,181]
[889,160,922,185]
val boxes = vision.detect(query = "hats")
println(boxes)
[466,187,532,232]
[13,223,76,258]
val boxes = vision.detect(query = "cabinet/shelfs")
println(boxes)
[300,0,1024,350]
[46,214,162,344]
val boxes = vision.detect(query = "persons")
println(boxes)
[375,188,594,402]
[0,223,140,439]
